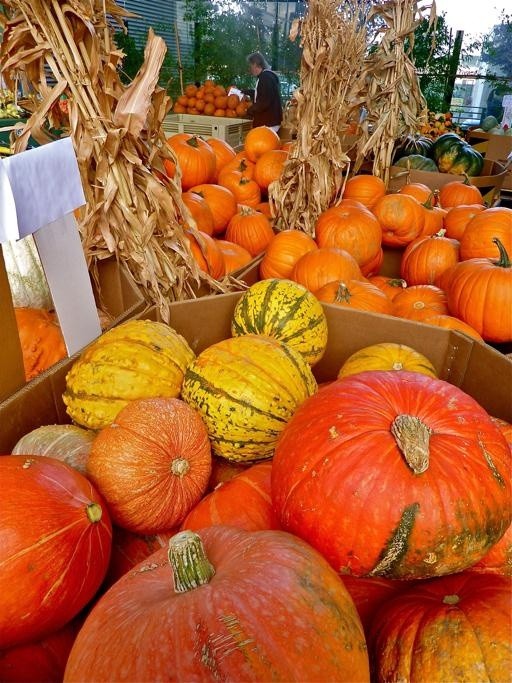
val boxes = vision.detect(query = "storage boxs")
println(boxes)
[1,127,511,458]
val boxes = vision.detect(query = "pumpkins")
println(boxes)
[1,80,512,683]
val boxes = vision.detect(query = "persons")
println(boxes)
[242,52,283,133]
